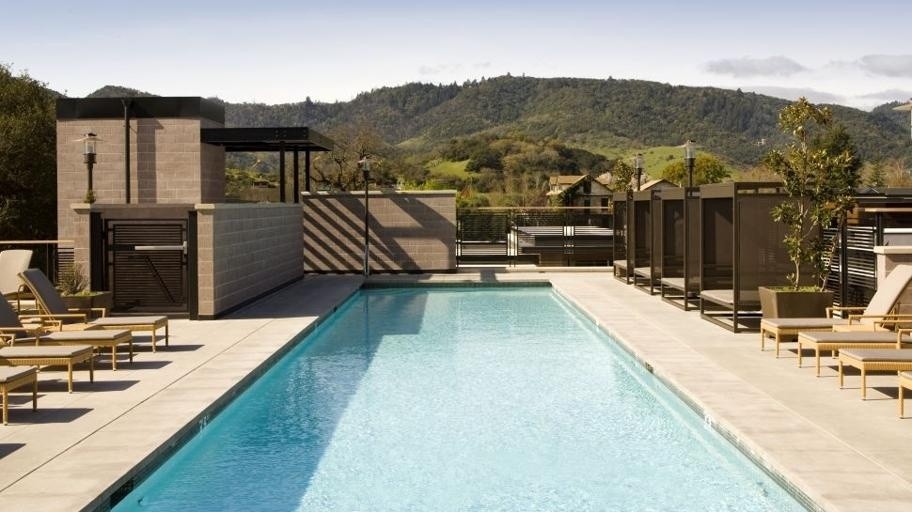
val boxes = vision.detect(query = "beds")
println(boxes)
[613,182,822,333]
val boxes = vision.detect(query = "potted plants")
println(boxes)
[758,97,864,342]
[38,262,114,327]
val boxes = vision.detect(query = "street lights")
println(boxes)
[676,136,704,186]
[72,131,106,202]
[632,150,644,191]
[356,154,373,275]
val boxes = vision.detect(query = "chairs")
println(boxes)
[838,349,912,401]
[760,264,912,358]
[797,320,912,377]
[0,365,38,426]
[0,291,133,371]
[17,268,168,353]
[0,334,93,394]
[898,371,912,419]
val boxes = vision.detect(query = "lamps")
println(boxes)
[636,153,644,191]
[685,139,696,196]
[83,133,97,202]
